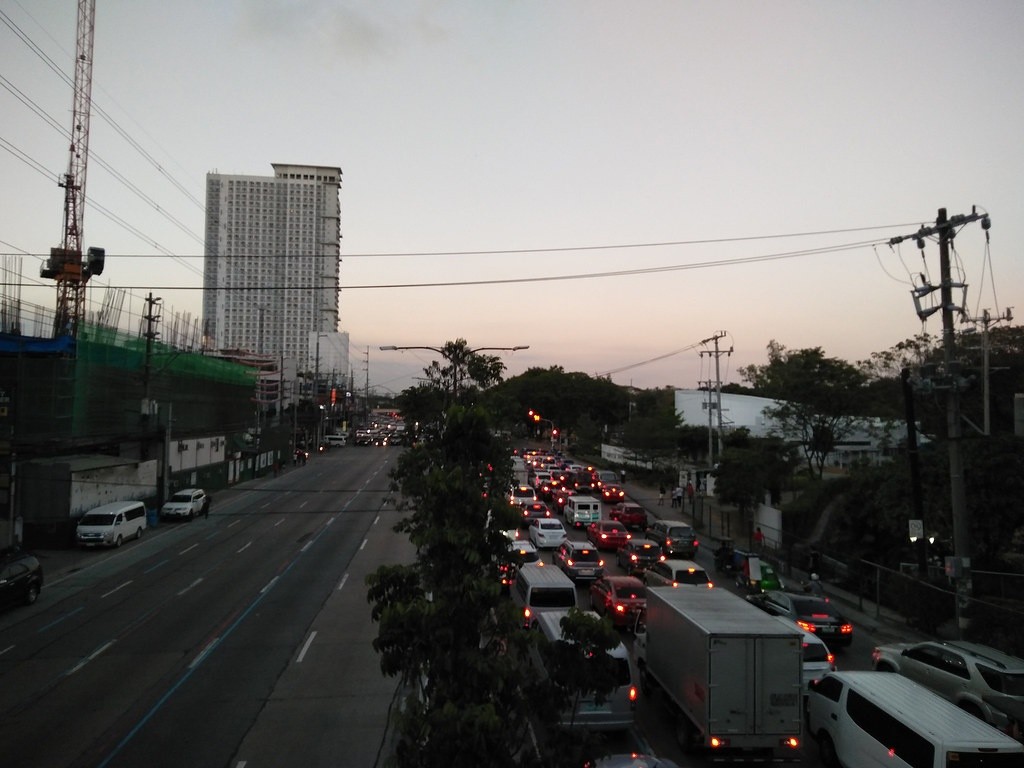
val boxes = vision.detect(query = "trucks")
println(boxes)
[633,585,805,761]
[563,496,602,530]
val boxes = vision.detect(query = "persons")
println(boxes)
[802,573,823,594]
[696,477,702,491]
[204,495,211,519]
[671,485,684,508]
[657,483,665,505]
[688,482,694,504]
[752,527,766,550]
[293,452,297,466]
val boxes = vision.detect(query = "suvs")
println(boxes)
[872,639,1024,731]
[503,501,713,623]
[0,548,44,614]
[160,489,206,522]
[773,616,836,696]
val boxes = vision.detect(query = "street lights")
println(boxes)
[380,345,529,404]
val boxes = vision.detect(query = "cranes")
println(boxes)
[42,0,104,339]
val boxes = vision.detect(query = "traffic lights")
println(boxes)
[528,410,540,421]
[553,429,557,438]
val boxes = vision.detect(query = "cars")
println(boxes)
[585,754,679,768]
[354,416,405,446]
[510,447,624,505]
[757,588,853,648]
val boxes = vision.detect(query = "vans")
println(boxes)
[808,670,1024,768]
[325,435,347,448]
[76,501,147,548]
[510,564,580,628]
[526,611,637,736]
